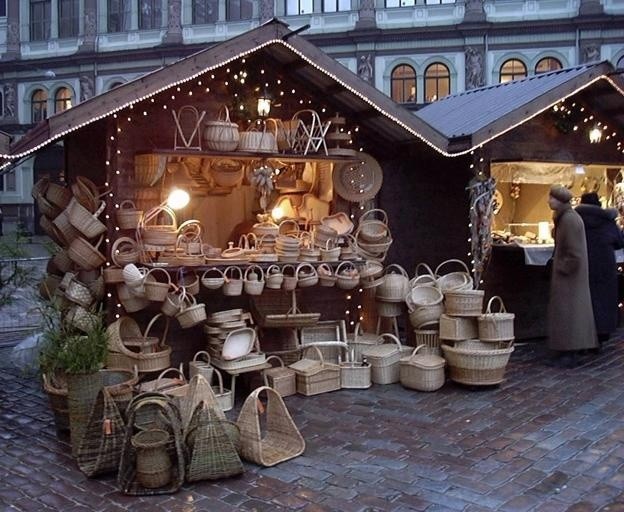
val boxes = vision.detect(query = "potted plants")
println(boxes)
[19,274,109,456]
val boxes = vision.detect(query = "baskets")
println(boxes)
[30,174,516,498]
[241,117,281,156]
[30,174,515,496]
[204,104,242,155]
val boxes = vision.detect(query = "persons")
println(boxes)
[541,183,600,368]
[79,75,93,101]
[465,46,483,90]
[581,43,600,65]
[356,54,373,84]
[573,191,624,345]
[3,82,16,119]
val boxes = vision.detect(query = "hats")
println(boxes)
[580,192,602,208]
[331,151,386,205]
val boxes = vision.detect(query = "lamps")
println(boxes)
[140,185,193,226]
[255,82,271,125]
